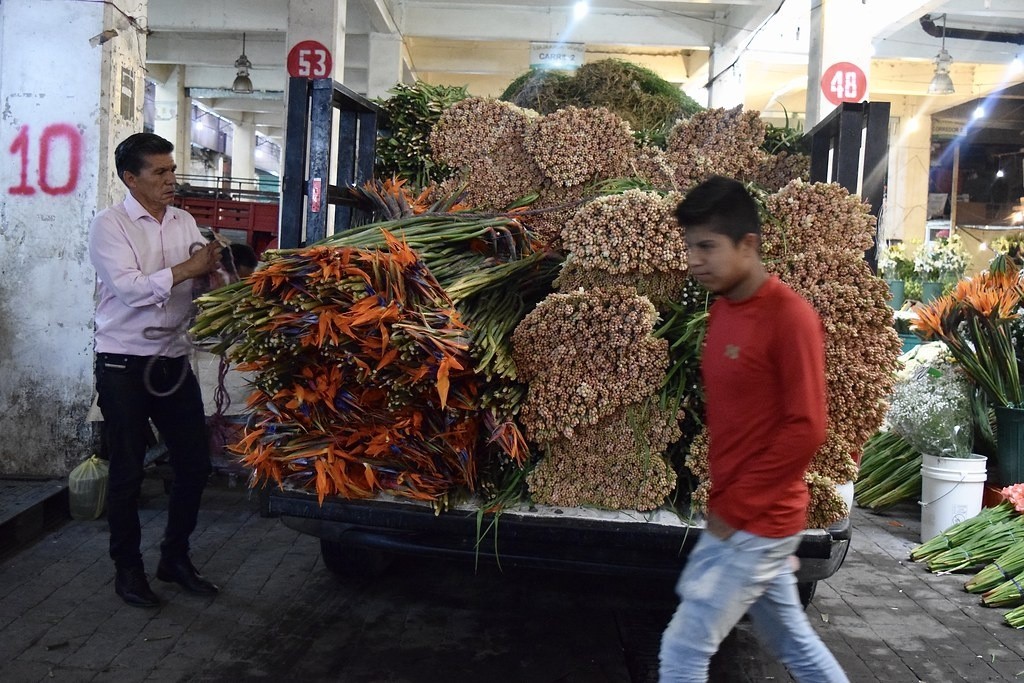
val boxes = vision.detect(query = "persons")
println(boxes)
[88,133,229,607]
[656,175,849,682]
[188,245,258,340]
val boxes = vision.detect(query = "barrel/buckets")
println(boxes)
[918,452,988,544]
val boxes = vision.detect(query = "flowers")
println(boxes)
[854,226,1024,631]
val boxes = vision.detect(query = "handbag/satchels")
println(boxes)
[67,454,109,522]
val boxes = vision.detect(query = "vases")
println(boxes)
[992,406,1024,488]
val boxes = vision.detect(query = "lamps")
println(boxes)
[232,32,254,94]
[926,14,955,94]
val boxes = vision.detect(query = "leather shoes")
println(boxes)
[115,567,160,607]
[155,554,219,596]
